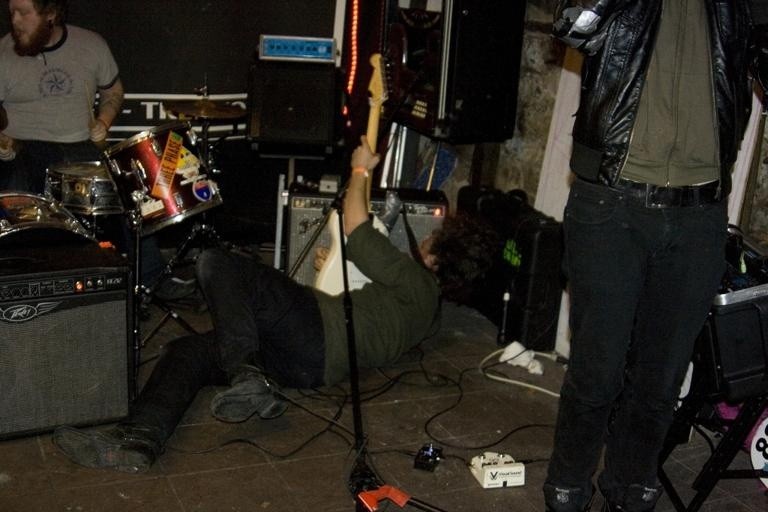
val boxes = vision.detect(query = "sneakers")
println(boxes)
[544,484,663,511]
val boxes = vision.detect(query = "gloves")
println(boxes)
[90,120,107,142]
[0,133,16,161]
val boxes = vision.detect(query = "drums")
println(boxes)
[96,118,224,237]
[44,162,125,215]
[0,191,107,276]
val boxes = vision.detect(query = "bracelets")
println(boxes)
[351,166,369,180]
[96,119,111,130]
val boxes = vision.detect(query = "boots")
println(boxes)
[51,418,170,474]
[210,366,288,423]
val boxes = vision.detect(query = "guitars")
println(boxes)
[313,54,389,295]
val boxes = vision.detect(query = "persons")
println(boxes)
[50,132,499,477]
[537,2,768,510]
[0,0,196,310]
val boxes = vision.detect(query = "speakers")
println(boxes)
[246,58,337,146]
[284,187,449,291]
[0,255,134,443]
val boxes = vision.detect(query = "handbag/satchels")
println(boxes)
[456,184,562,351]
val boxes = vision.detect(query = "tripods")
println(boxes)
[132,206,198,352]
[139,122,263,324]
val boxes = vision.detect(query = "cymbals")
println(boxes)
[163,99,247,119]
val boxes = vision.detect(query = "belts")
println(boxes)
[614,177,720,208]
